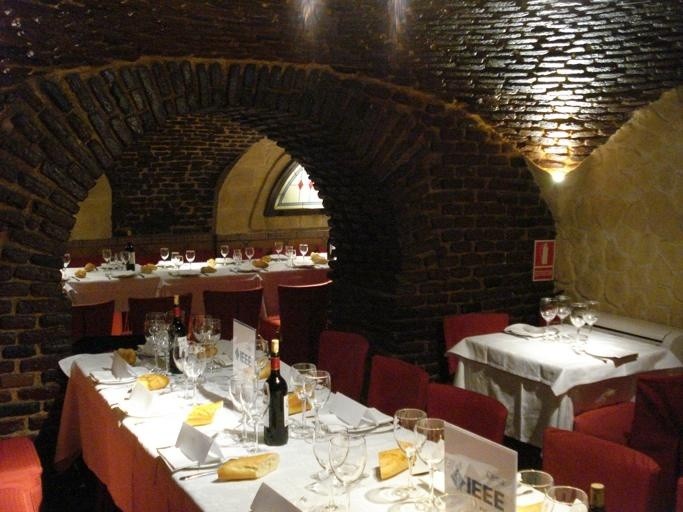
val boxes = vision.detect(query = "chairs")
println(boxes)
[316,329,369,400]
[444,313,681,510]
[58,235,331,362]
[0,437,44,510]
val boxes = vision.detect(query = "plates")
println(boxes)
[88,365,149,385]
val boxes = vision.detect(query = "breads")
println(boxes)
[260,362,271,378]
[216,454,278,482]
[310,251,326,265]
[85,263,96,272]
[187,400,223,425]
[379,448,409,480]
[141,263,156,275]
[139,373,170,391]
[253,260,269,269]
[196,343,217,359]
[260,255,272,263]
[288,391,310,415]
[207,258,216,267]
[117,346,137,364]
[75,269,86,278]
[200,267,217,274]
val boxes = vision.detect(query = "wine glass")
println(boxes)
[142,292,604,511]
[60,229,312,282]
